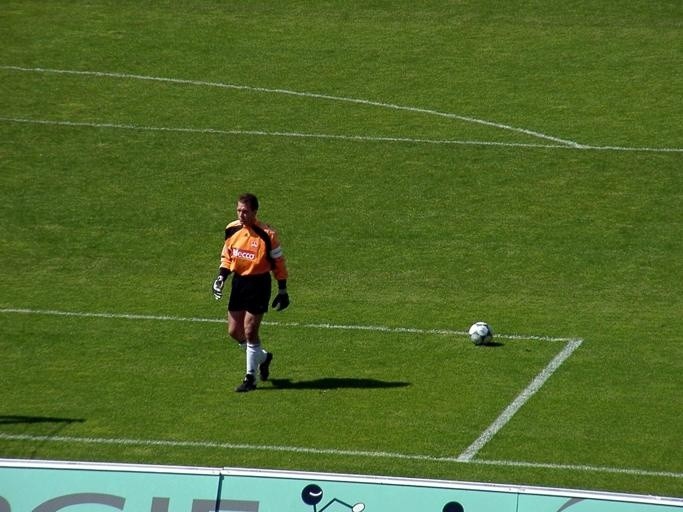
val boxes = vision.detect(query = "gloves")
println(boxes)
[212,275,226,301]
[272,285,290,311]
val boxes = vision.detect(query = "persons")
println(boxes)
[212,192,290,392]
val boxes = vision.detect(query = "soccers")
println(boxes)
[469,322,492,345]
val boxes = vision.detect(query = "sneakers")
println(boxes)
[259,352,272,381]
[234,374,257,392]
